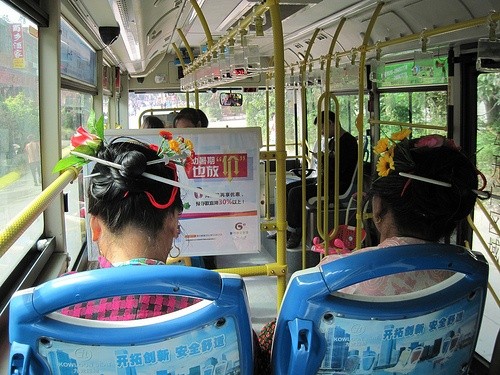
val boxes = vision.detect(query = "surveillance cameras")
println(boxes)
[99,26,120,46]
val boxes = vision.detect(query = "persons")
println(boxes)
[140,116,164,130]
[127,93,192,116]
[173,107,208,128]
[267,111,358,248]
[53,136,260,375]
[24,135,42,185]
[221,94,242,105]
[253,132,492,375]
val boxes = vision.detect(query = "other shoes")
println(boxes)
[286,232,302,250]
[266,230,291,240]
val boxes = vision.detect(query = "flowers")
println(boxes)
[310,237,352,255]
[372,130,462,178]
[51,108,107,175]
[157,131,197,166]
[219,91,244,107]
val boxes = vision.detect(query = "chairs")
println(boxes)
[285,135,368,212]
[270,244,489,375]
[9,244,255,375]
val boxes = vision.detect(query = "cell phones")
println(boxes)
[309,151,314,153]
